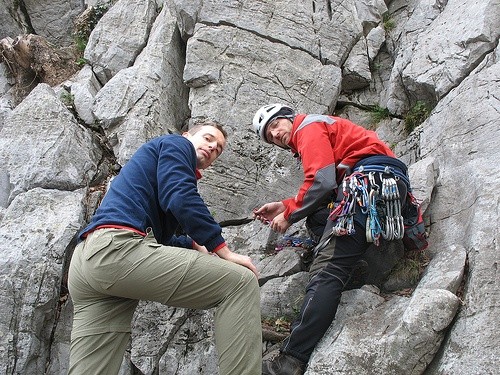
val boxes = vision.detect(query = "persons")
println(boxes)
[67,124,262,375]
[253,103,428,375]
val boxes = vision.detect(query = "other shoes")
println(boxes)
[261,352,308,375]
[346,259,368,291]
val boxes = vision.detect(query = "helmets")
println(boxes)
[252,103,295,150]
